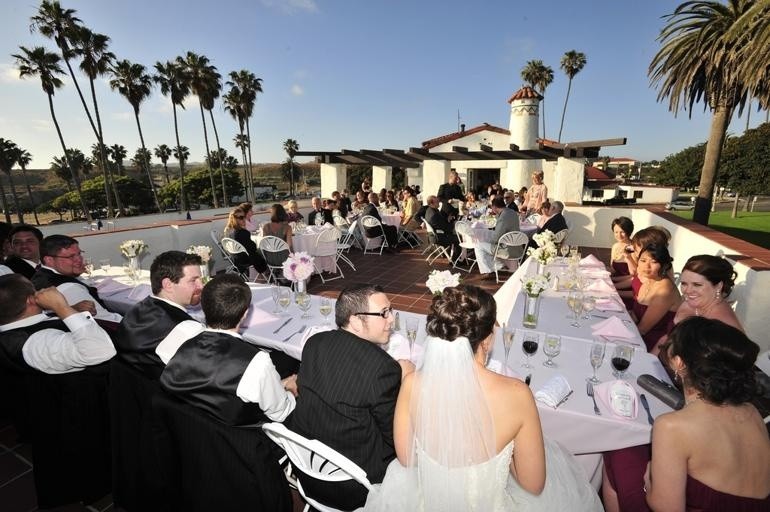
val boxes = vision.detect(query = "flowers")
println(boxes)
[524,276,547,295]
[283,252,313,281]
[185,245,213,262]
[120,240,144,258]
[426,270,462,295]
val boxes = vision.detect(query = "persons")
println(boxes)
[602,316,770,512]
[223,179,421,286]
[2,222,297,433]
[615,226,672,302]
[416,169,568,270]
[650,254,745,391]
[609,216,634,284]
[285,283,416,511]
[352,283,604,511]
[624,243,683,352]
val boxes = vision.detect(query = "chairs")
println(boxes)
[263,423,379,512]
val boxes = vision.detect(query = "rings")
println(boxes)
[643,486,647,493]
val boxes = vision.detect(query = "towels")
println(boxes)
[592,314,641,347]
[93,275,133,296]
[577,253,626,312]
[594,377,640,420]
[534,373,573,409]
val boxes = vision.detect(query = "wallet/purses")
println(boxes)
[636,372,685,410]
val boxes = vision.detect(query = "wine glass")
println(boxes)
[80,244,657,426]
[350,203,404,217]
[455,198,537,227]
[253,217,324,235]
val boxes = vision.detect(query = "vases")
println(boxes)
[522,295,542,329]
[295,280,307,300]
[126,258,141,274]
[200,262,210,284]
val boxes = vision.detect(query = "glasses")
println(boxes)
[354,306,394,319]
[45,250,85,260]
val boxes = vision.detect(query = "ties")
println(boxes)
[35,264,41,272]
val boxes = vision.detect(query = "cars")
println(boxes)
[666,202,695,210]
[258,191,320,200]
[723,189,742,198]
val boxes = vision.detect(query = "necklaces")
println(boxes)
[695,308,699,316]
[644,279,650,296]
[683,397,697,408]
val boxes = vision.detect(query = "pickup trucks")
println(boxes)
[604,196,637,205]
